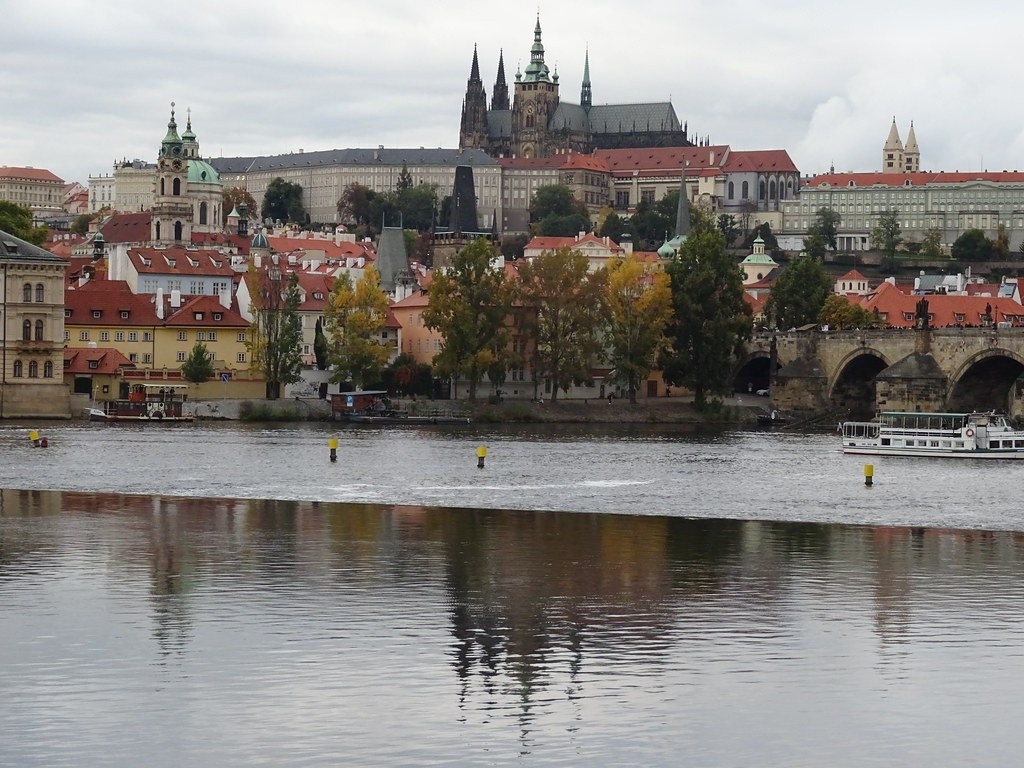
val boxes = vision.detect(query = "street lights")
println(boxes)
[785,306,787,332]
[994,304,998,328]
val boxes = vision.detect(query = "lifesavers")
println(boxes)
[967,429,974,437]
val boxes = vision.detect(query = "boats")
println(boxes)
[840,411,1024,459]
[84,383,194,422]
